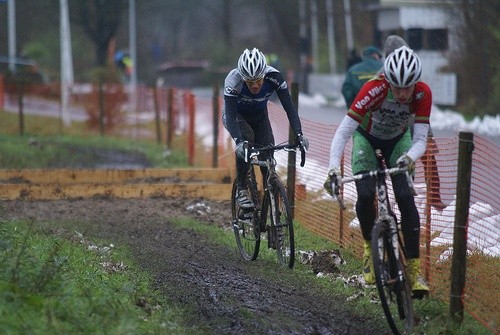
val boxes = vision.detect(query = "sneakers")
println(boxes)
[362,239,378,285]
[237,185,256,210]
[406,258,430,295]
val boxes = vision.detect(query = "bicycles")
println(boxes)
[327,150,416,335]
[231,139,306,271]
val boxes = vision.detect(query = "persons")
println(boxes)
[221,47,309,250]
[341,47,385,110]
[345,49,362,71]
[323,46,432,299]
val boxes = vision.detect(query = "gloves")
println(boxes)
[397,155,415,178]
[299,135,309,150]
[324,169,342,197]
[237,143,250,160]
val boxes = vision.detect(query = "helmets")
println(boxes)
[384,46,422,88]
[238,47,266,83]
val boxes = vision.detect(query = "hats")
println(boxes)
[385,36,411,58]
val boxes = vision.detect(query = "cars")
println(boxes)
[0,57,43,87]
